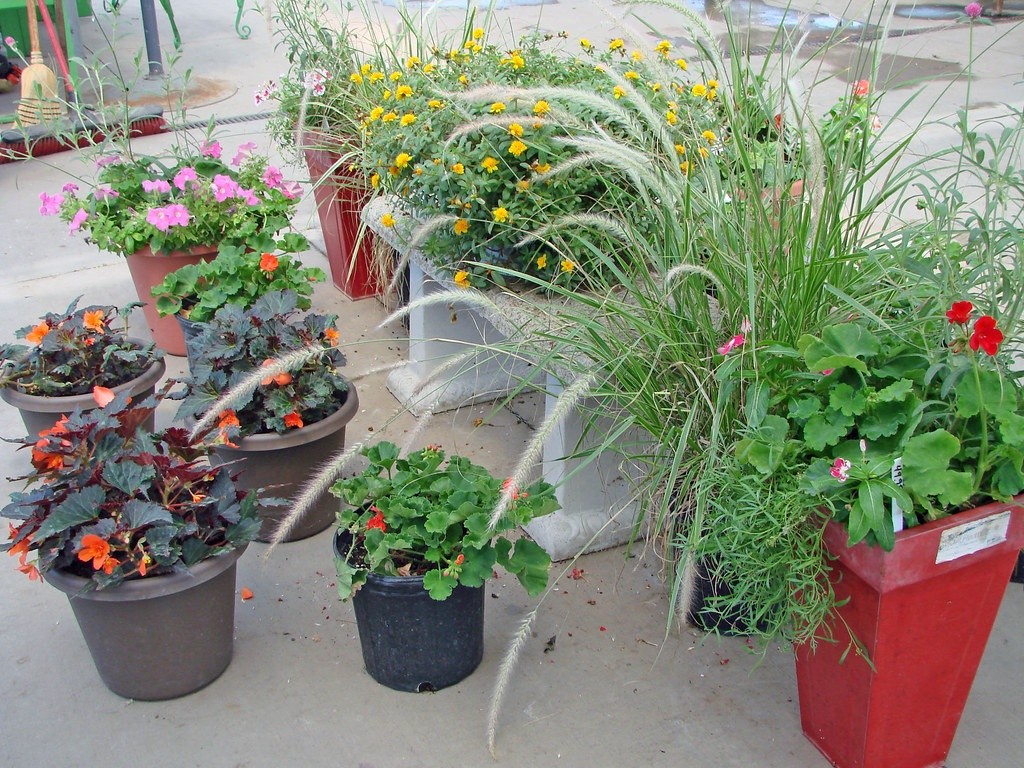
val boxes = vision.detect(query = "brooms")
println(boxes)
[0,0,169,164]
[12,0,62,130]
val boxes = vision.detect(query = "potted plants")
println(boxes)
[188,1,1023,755]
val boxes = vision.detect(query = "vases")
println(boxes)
[333,501,485,694]
[40,533,238,697]
[185,372,359,543]
[296,122,386,302]
[174,293,212,371]
[0,338,166,434]
[121,244,256,356]
[791,493,1023,768]
[739,181,807,257]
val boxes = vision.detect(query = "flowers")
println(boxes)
[325,443,562,599]
[150,248,326,319]
[0,295,174,394]
[244,0,401,152]
[719,302,1023,555]
[0,386,292,602]
[0,15,304,254]
[166,289,348,449]
[341,35,726,287]
[695,102,813,185]
